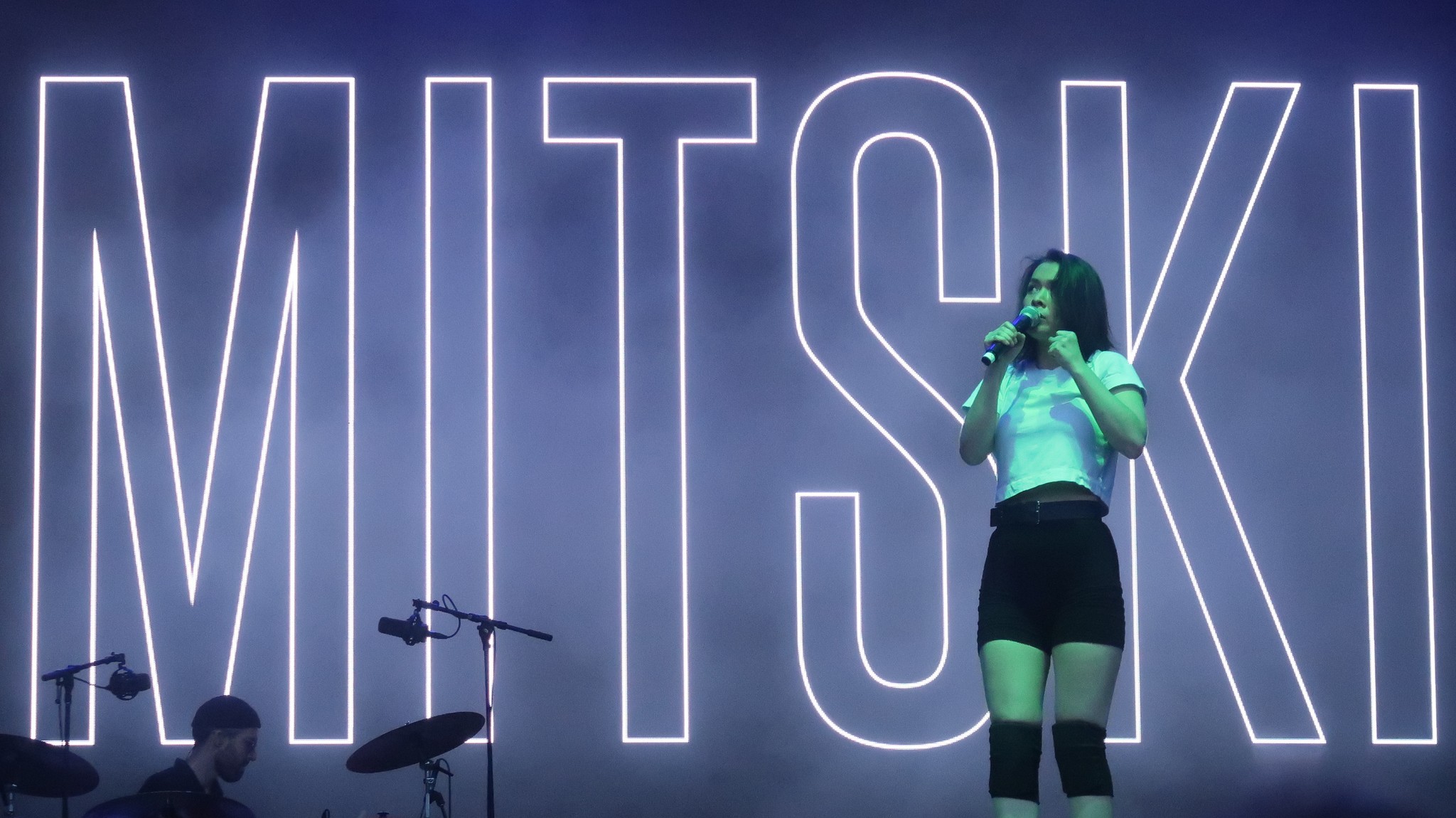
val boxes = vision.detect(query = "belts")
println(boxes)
[990,500,1103,527]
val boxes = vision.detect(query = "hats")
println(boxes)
[194,695,261,729]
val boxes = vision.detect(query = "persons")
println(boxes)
[138,693,263,818]
[957,245,1146,818]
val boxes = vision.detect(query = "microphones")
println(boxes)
[379,617,449,647]
[981,307,1041,365]
[106,666,151,701]
[429,759,441,803]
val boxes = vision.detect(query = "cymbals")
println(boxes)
[85,792,255,818]
[346,712,485,774]
[0,734,100,799]
